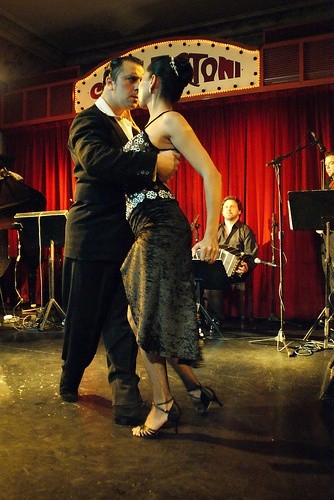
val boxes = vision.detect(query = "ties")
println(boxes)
[132,127,140,137]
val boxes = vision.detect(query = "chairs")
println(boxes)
[211,271,255,331]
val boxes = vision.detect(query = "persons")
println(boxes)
[321,153,333,291]
[119,54,223,436]
[209,197,257,334]
[59,57,180,424]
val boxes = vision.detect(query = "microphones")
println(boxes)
[253,257,278,267]
[310,131,326,152]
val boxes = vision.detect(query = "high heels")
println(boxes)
[133,396,182,439]
[186,382,223,415]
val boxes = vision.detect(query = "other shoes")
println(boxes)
[209,319,223,334]
[60,382,78,402]
[114,404,179,428]
[304,320,325,330]
[330,320,334,332]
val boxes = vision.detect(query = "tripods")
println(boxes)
[191,258,231,342]
[14,214,68,332]
[247,141,315,357]
[287,188,334,350]
